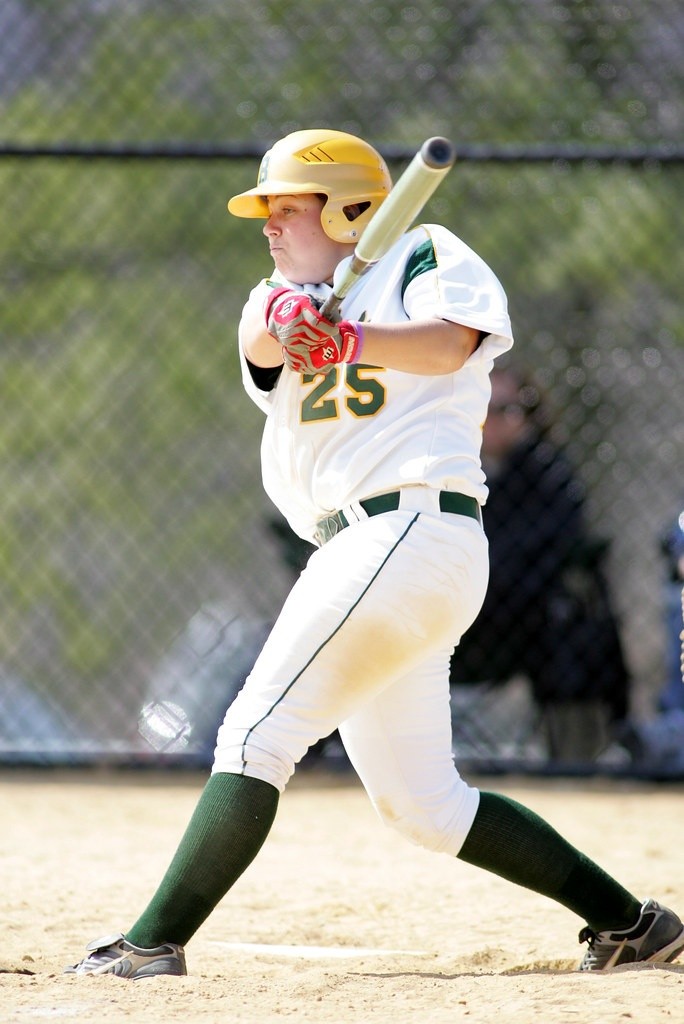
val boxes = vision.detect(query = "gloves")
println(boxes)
[281,320,364,376]
[261,287,339,357]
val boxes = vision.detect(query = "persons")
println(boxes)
[62,129,684,982]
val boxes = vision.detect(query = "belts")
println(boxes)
[313,490,482,546]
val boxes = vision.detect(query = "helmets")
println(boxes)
[227,128,392,243]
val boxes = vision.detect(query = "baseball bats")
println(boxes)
[319,135,457,322]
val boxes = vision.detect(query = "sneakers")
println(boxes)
[63,932,188,981]
[579,897,684,970]
